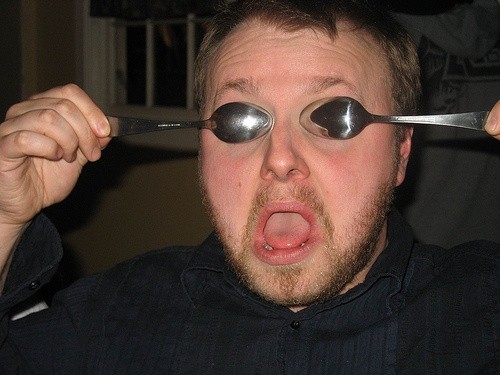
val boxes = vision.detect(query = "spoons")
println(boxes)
[299,96,490,140]
[104,101,271,144]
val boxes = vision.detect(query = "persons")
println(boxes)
[0,1,499,375]
